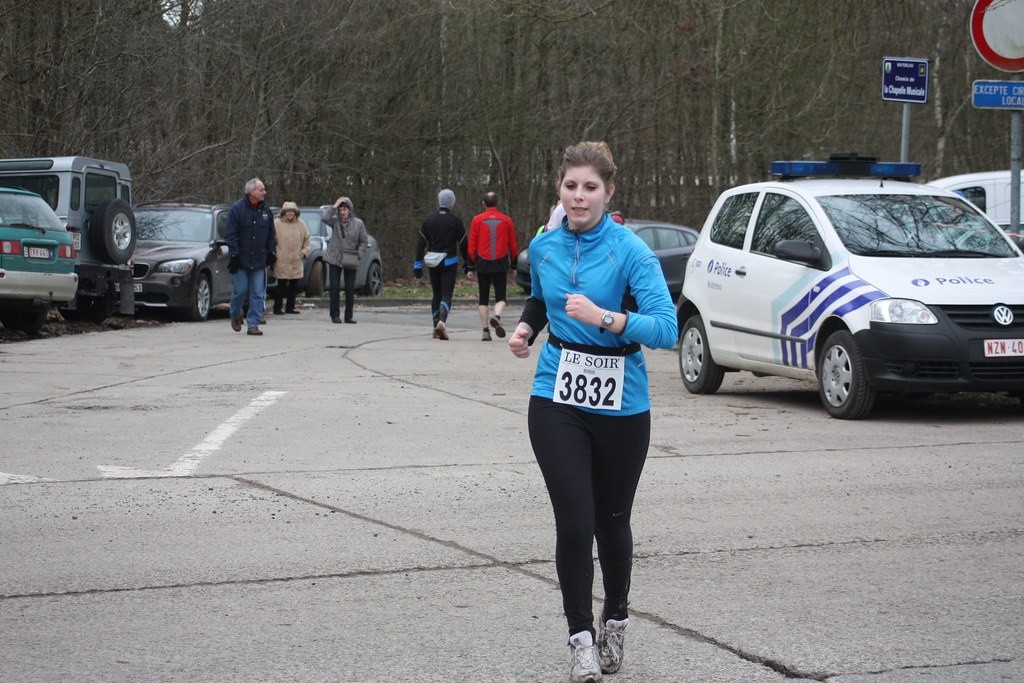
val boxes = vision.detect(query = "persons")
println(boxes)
[414,189,468,340]
[272,201,312,315]
[224,178,276,335]
[467,192,518,341]
[509,141,678,683]
[321,196,368,324]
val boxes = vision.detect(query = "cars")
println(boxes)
[0,184,79,335]
[673,151,1024,420]
[106,198,241,323]
[514,218,700,303]
[269,204,382,299]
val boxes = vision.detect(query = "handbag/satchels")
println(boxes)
[423,251,447,268]
[341,248,359,270]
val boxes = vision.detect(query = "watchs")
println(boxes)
[599,310,614,334]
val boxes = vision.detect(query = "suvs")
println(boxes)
[0,155,137,323]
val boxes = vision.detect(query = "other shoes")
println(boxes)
[286,309,300,314]
[345,318,356,324]
[433,331,440,338]
[333,316,341,323]
[435,321,450,340]
[259,318,266,324]
[273,310,285,315]
[231,315,241,331]
[247,326,263,335]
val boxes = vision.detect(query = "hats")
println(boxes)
[438,189,455,209]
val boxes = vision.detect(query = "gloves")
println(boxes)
[462,263,471,273]
[414,268,424,279]
[267,253,277,271]
[226,254,240,274]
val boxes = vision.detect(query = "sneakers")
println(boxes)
[482,331,492,341]
[490,317,506,337]
[597,616,629,673]
[566,631,602,683]
